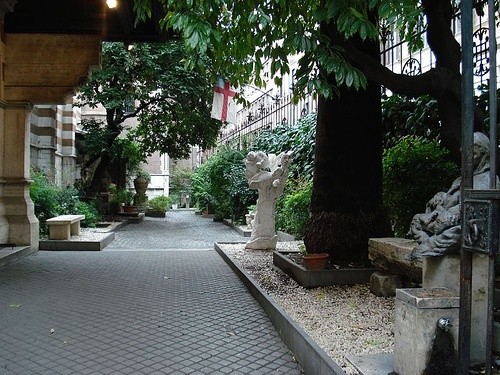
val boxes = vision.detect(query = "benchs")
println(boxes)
[367,237,425,297]
[45,215,86,240]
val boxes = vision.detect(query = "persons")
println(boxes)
[405,131,500,262]
[280,154,290,169]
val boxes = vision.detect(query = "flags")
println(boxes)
[210,75,237,126]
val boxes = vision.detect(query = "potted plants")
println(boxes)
[143,194,173,218]
[134,170,151,196]
[296,210,336,271]
[124,203,136,213]
[169,194,181,209]
